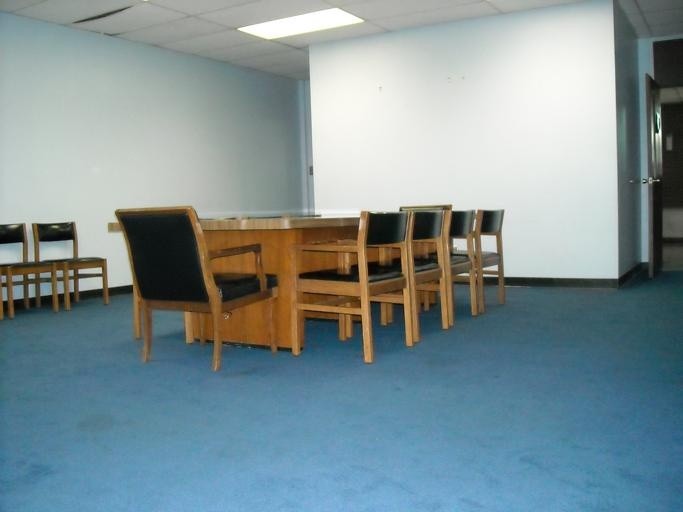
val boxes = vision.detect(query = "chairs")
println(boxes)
[288,207,415,367]
[113,204,281,374]
[344,204,452,347]
[416,203,481,327]
[1,221,109,320]
[423,204,506,314]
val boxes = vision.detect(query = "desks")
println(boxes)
[109,214,362,350]
